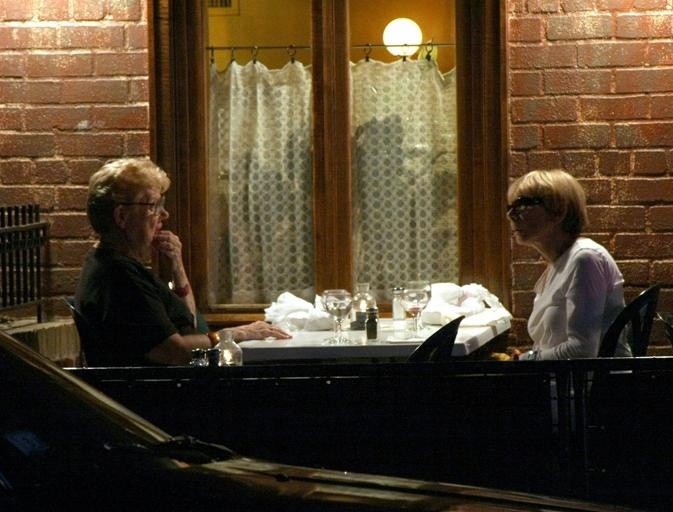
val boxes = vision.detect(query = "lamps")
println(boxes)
[381,16,422,58]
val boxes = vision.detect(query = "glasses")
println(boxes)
[116,196,166,215]
[504,194,541,212]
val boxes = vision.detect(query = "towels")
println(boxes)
[264,289,335,332]
[420,282,515,327]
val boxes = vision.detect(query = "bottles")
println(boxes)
[363,307,380,340]
[349,283,378,330]
[189,347,221,369]
[213,329,242,368]
[388,287,405,321]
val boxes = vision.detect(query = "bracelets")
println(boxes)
[205,329,221,346]
[511,347,522,362]
[167,279,191,297]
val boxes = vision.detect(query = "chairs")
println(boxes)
[60,294,111,368]
[585,284,662,433]
[399,315,469,365]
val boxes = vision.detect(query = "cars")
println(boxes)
[0,327,617,511]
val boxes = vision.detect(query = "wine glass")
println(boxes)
[320,289,352,345]
[391,277,432,339]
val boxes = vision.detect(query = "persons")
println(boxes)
[487,168,634,376]
[72,155,293,368]
